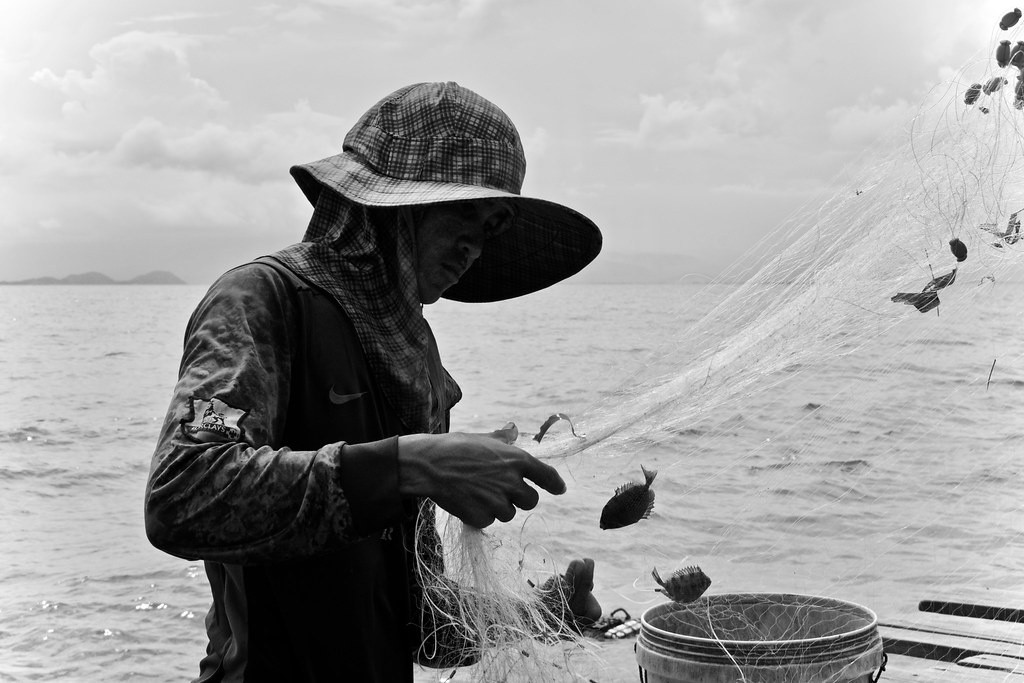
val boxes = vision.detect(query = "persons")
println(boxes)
[143,79,601,683]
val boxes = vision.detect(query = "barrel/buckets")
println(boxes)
[636,593,884,683]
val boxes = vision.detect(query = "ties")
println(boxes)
[288,80,605,305]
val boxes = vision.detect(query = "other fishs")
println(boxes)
[599,463,659,531]
[651,564,713,604]
